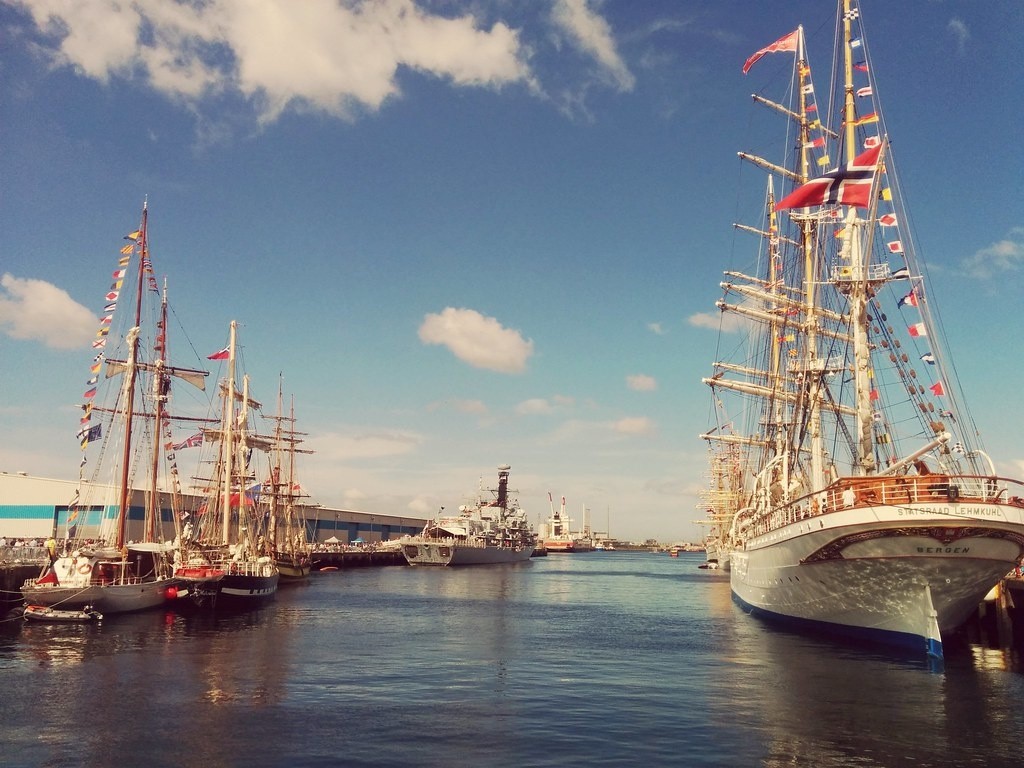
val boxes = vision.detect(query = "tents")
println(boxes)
[351,537,368,548]
[324,536,343,546]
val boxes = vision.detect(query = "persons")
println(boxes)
[788,485,857,522]
[1,535,143,564]
[312,543,372,552]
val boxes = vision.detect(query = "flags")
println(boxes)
[764,4,965,453]
[34,557,59,585]
[173,431,203,450]
[206,344,230,360]
[743,29,799,78]
[64,226,187,544]
[773,143,882,214]
[194,447,300,518]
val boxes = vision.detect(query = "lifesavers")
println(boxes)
[231,564,237,573]
[28,606,49,612]
[78,563,91,574]
[504,541,510,547]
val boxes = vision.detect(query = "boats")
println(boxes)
[399,464,539,566]
[24,604,103,622]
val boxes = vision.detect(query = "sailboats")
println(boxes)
[701,0,1024,658]
[20,191,323,621]
